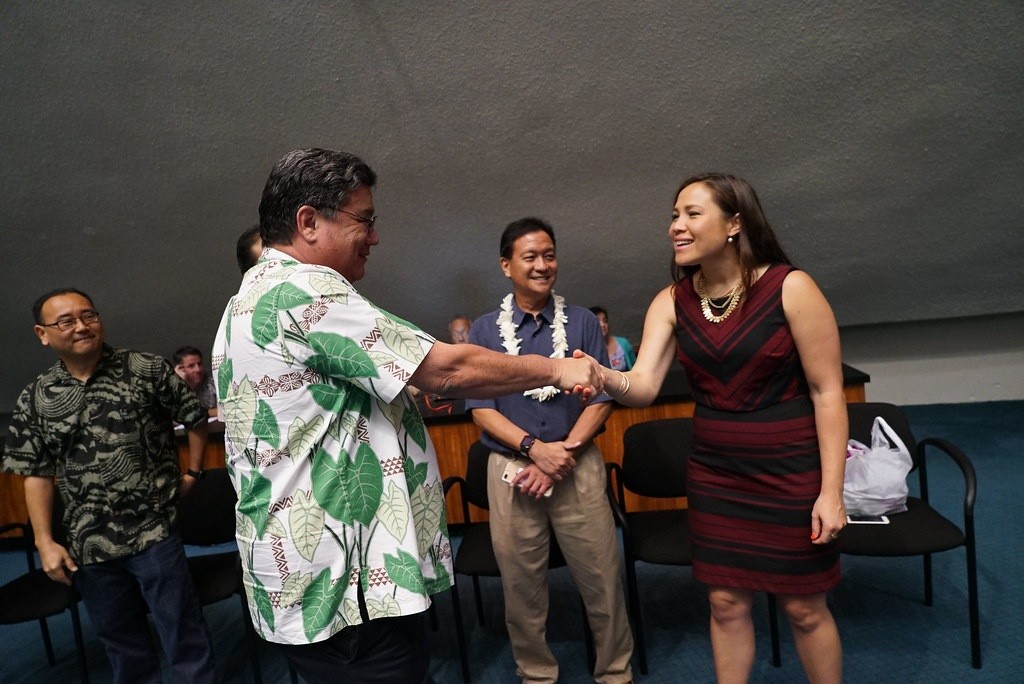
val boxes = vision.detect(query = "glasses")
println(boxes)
[315,203,378,234]
[44,311,99,332]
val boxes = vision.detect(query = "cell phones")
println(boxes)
[847,515,889,524]
[501,461,554,497]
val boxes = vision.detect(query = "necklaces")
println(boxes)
[499,290,569,401]
[697,265,748,322]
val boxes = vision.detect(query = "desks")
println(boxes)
[0,358,870,553]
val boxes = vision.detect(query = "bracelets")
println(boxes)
[602,374,626,396]
[604,369,629,400]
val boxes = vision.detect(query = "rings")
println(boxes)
[831,533,838,538]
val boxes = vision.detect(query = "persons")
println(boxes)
[565,172,850,684]
[4,216,635,684]
[212,149,608,684]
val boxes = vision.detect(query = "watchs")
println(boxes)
[185,469,204,480]
[519,432,536,456]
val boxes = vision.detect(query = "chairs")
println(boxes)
[839,402,981,669]
[0,486,91,684]
[603,418,783,675]
[441,438,596,684]
[137,468,298,684]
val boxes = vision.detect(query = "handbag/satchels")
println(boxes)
[844,416,914,517]
